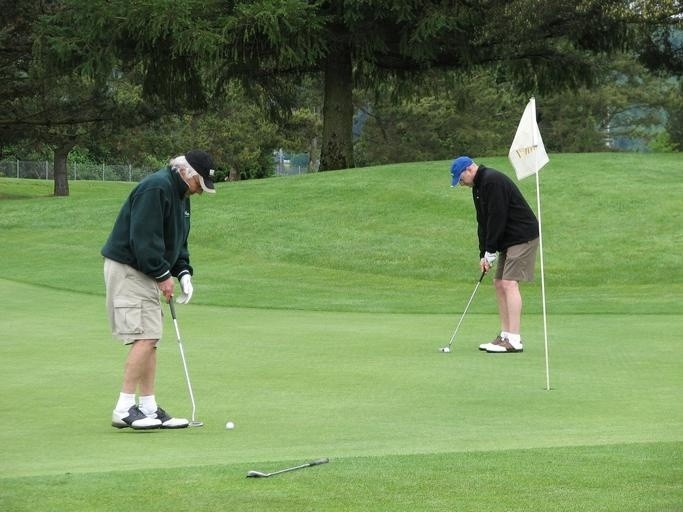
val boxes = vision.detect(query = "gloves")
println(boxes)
[483,250,499,269]
[173,271,196,306]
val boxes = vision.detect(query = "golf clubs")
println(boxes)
[438,268,487,351]
[246,457,329,478]
[168,296,204,427]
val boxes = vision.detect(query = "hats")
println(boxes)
[183,147,219,196]
[447,154,474,189]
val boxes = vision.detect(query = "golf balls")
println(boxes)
[226,422,235,429]
[444,347,450,353]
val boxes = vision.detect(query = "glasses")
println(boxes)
[190,173,202,191]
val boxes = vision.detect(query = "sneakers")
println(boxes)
[485,336,525,354]
[109,404,163,430]
[135,402,190,429]
[478,334,507,351]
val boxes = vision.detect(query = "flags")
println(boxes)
[507,98,550,180]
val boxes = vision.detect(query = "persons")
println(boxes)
[450,156,539,353]
[100,150,217,429]
